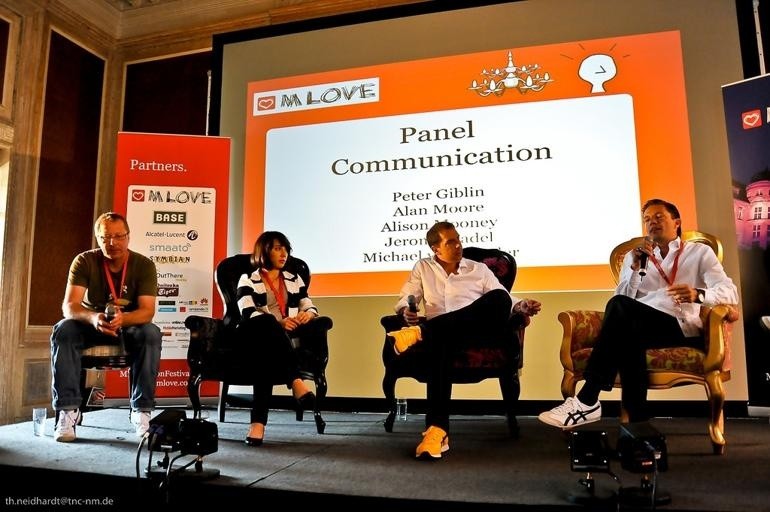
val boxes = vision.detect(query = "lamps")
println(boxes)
[142,407,221,482]
[567,422,672,506]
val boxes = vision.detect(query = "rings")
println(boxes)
[636,247,642,252]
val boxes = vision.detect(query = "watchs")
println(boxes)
[692,287,705,305]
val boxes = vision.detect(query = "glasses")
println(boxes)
[100,231,128,242]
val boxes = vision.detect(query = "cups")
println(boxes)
[33,407,47,436]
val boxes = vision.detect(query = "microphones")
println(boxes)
[104,306,115,322]
[640,236,655,275]
[408,294,417,314]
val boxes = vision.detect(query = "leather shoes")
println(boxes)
[293,389,314,405]
[245,426,264,446]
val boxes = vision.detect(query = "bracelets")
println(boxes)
[517,300,523,313]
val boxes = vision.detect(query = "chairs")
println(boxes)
[558,231,739,456]
[379,246,531,441]
[184,253,334,435]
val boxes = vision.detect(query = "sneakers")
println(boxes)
[131,409,151,438]
[387,325,423,356]
[539,396,601,428]
[416,425,449,459]
[55,408,81,441]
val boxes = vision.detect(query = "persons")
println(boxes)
[537,199,741,461]
[231,231,320,447]
[48,210,162,442]
[385,222,542,462]
